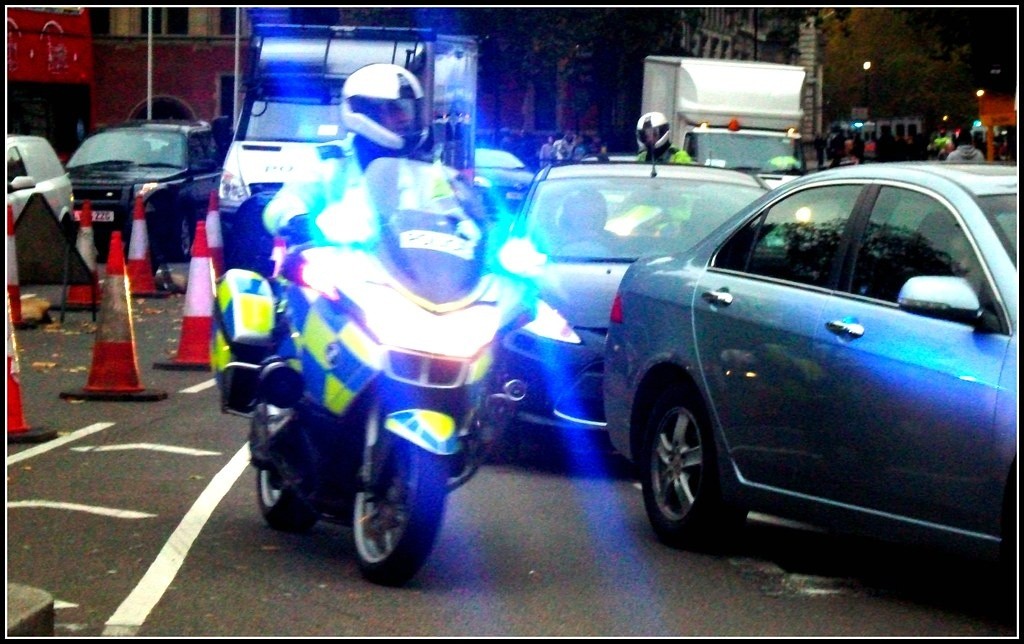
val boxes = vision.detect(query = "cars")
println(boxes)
[7,130,76,248]
[483,163,775,463]
[473,142,537,215]
[599,159,1016,620]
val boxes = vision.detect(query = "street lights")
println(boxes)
[862,61,874,120]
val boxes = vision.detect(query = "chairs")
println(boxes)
[864,212,983,305]
[125,141,151,161]
[669,196,745,256]
[549,190,624,256]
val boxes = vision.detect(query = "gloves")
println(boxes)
[280,213,314,246]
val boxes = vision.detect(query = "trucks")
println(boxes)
[215,18,483,250]
[639,52,810,189]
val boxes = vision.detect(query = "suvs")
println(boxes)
[64,118,225,264]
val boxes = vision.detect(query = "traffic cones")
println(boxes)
[57,229,171,403]
[8,290,58,446]
[127,193,172,301]
[6,202,39,330]
[47,200,105,313]
[149,219,217,373]
[265,236,288,279]
[203,186,225,282]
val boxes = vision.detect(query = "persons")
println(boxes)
[636,111,695,237]
[565,189,607,239]
[908,212,973,276]
[928,127,1008,162]
[251,64,465,473]
[814,125,865,170]
[500,127,606,167]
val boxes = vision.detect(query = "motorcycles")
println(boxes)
[206,157,547,590]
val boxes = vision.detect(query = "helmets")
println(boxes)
[340,64,428,156]
[637,111,670,149]
